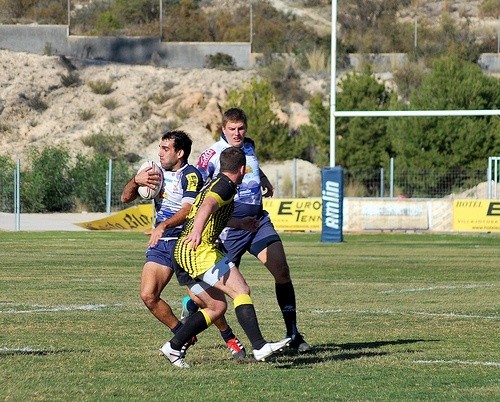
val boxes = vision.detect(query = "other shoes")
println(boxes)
[285,335,311,351]
[252,338,291,361]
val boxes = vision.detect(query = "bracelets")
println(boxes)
[260,175,266,180]
[133,176,139,186]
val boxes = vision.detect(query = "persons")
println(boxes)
[121,130,246,362]
[158,146,292,368]
[180,108,312,353]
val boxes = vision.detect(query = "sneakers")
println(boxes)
[222,334,246,360]
[160,342,190,370]
[181,296,200,320]
[159,342,194,369]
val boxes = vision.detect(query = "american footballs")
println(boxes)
[136,161,163,200]
